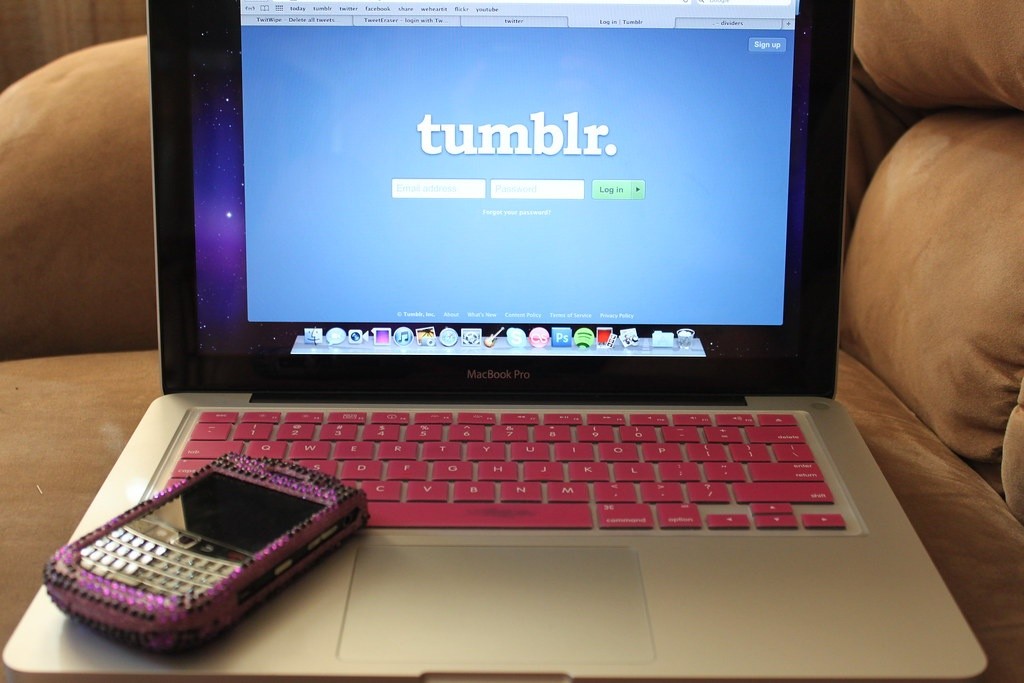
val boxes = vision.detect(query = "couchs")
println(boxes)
[0,0,1024,683]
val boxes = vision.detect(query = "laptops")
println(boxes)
[1,0,989,683]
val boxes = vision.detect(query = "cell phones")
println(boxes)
[43,451,369,656]
